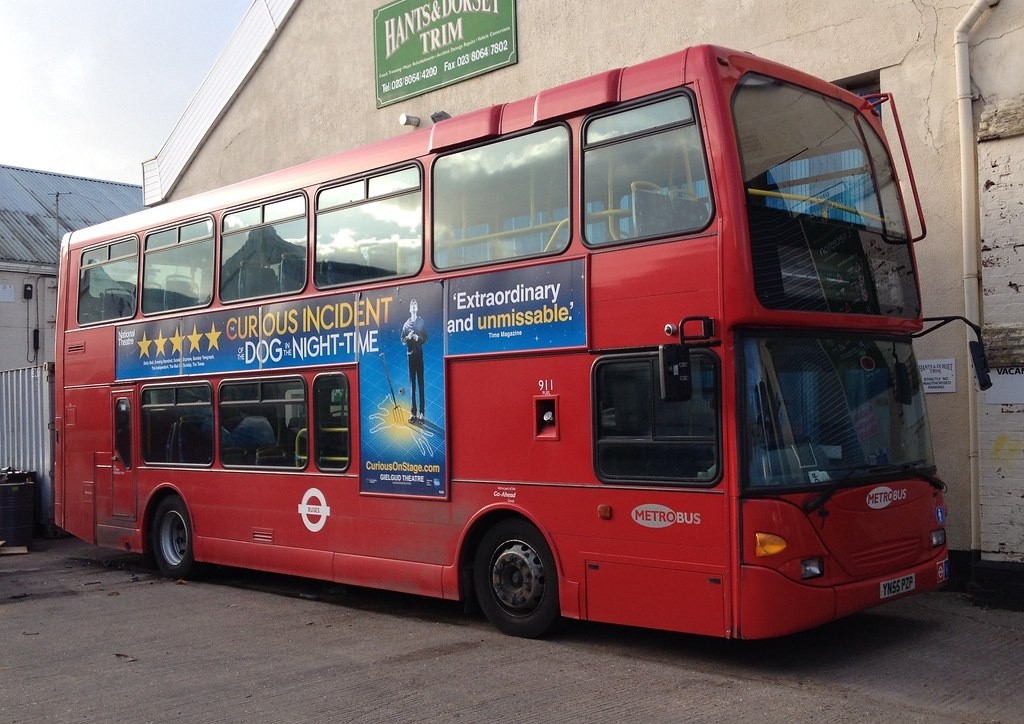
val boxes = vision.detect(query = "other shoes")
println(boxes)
[418,417,425,425]
[408,416,415,422]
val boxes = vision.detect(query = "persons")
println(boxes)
[401,299,427,425]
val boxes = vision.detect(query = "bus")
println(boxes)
[52,42,993,641]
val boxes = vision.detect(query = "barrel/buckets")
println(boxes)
[0,483,39,543]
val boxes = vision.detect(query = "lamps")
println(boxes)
[431,111,451,124]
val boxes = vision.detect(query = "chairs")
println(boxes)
[254,447,283,466]
[666,188,708,231]
[101,289,132,320]
[628,180,676,238]
[222,446,247,465]
[133,239,419,315]
